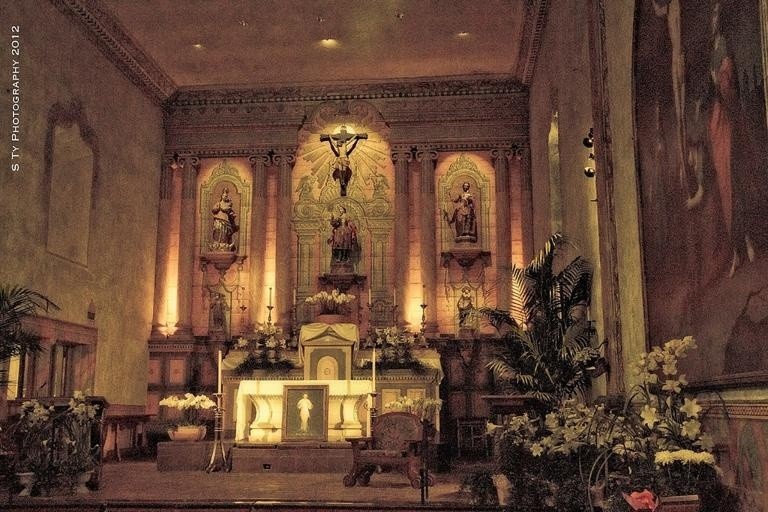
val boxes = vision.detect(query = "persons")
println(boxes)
[456,288,475,319]
[210,292,229,329]
[296,393,313,432]
[212,192,234,246]
[448,181,477,236]
[329,133,358,186]
[330,206,357,263]
[640,1,759,278]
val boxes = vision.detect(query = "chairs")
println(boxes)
[342,411,436,488]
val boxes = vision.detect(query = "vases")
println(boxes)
[15,468,94,496]
[167,424,207,441]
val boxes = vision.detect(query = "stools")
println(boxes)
[456,417,491,456]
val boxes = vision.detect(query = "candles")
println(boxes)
[368,288,372,305]
[423,285,425,305]
[393,288,397,305]
[217,349,222,394]
[371,347,376,393]
[269,288,272,306]
[293,288,296,306]
[242,287,245,307]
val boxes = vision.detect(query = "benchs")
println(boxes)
[105,403,158,463]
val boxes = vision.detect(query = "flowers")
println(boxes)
[304,290,357,314]
[232,331,295,377]
[484,338,727,512]
[385,395,444,423]
[367,325,429,374]
[11,387,106,473]
[159,393,218,425]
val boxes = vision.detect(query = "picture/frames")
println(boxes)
[282,384,329,442]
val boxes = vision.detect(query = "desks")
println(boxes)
[481,394,537,426]
